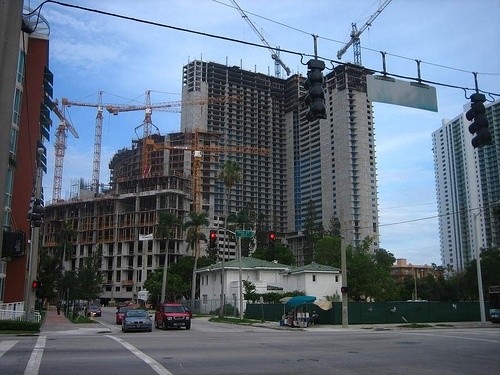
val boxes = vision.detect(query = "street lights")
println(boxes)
[415,269,423,300]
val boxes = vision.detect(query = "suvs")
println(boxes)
[155,303,192,331]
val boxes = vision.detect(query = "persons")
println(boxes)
[56,302,61,315]
[307,311,317,325]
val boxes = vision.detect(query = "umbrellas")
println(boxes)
[279,295,316,306]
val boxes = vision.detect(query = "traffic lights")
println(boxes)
[32,282,41,289]
[210,230,217,248]
[341,286,349,294]
[268,230,276,246]
[31,197,42,228]
[304,72,326,121]
[465,102,494,148]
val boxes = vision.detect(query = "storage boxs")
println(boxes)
[284,319,288,324]
[280,320,284,327]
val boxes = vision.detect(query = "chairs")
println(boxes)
[307,314,319,326]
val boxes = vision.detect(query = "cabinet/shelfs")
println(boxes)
[297,312,310,328]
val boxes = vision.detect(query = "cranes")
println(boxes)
[52,88,272,258]
[337,0,392,66]
[231,0,292,77]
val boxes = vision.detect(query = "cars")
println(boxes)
[85,305,103,318]
[77,304,84,311]
[116,307,153,332]
[66,302,72,307]
